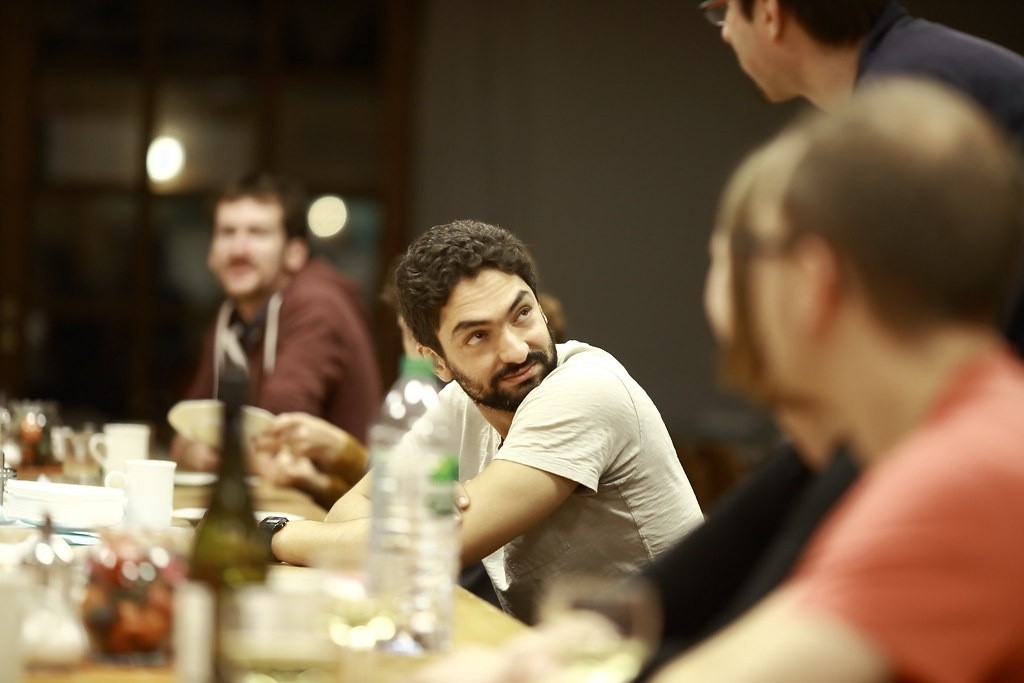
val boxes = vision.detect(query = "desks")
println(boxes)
[0,442,535,683]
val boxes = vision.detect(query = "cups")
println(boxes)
[105,459,178,531]
[89,424,150,488]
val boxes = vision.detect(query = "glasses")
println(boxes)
[699,0,731,26]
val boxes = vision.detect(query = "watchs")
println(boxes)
[259,515,289,563]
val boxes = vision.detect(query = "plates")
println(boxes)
[173,471,218,486]
[168,399,277,446]
[172,507,305,528]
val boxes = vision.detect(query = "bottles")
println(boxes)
[186,372,271,584]
[369,357,460,652]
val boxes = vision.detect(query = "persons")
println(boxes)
[254,218,708,623]
[250,252,451,504]
[168,164,381,473]
[644,79,1024,683]
[700,0,1024,146]
